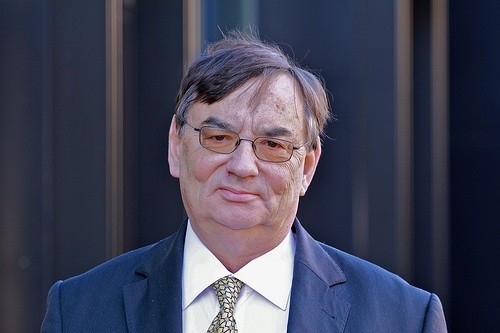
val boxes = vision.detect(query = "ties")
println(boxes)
[198,275,245,332]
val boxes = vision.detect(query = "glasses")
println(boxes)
[176,116,308,165]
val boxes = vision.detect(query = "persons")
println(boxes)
[39,27,450,332]
